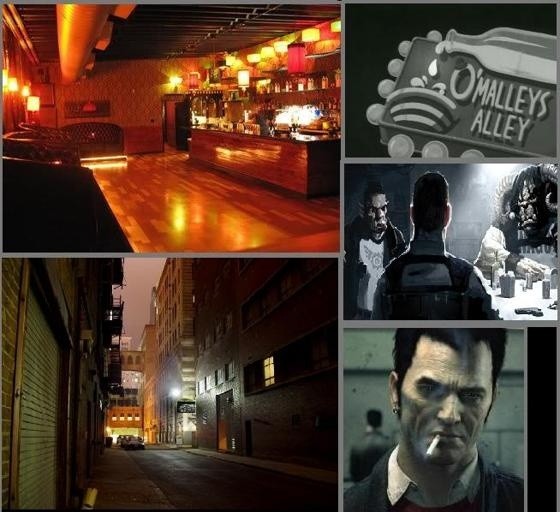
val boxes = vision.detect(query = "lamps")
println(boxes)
[285,29,307,76]
[237,69,251,93]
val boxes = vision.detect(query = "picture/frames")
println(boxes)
[30,82,55,107]
[65,101,111,118]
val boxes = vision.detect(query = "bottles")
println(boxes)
[490,248,506,289]
[550,268,559,289]
[259,71,341,93]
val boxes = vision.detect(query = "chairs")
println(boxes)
[3,122,80,166]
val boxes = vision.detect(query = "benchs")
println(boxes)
[60,122,127,163]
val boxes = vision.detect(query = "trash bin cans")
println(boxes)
[106,437,113,448]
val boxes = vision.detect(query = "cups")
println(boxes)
[542,280,551,299]
[525,272,533,289]
[496,273,517,299]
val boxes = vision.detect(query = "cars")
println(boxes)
[116,435,144,449]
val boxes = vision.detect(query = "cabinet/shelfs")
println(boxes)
[252,70,341,131]
[220,75,265,104]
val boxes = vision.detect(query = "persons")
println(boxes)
[342,327,524,512]
[370,170,503,320]
[342,181,409,320]
[474,227,546,281]
[349,408,395,488]
